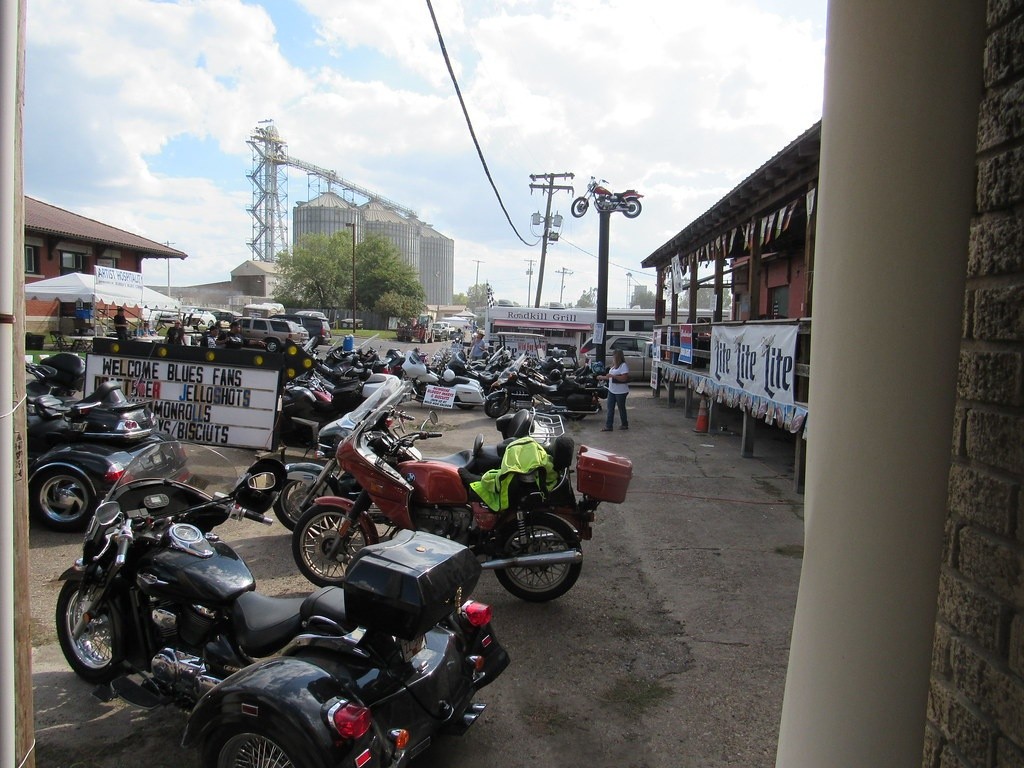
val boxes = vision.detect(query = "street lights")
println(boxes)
[346,222,356,333]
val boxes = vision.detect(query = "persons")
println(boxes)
[206,325,230,348]
[472,326,480,341]
[470,333,485,361]
[225,322,267,349]
[165,319,188,346]
[451,328,465,342]
[597,349,631,432]
[113,306,128,341]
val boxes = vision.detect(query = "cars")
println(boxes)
[396,314,455,344]
[154,302,332,354]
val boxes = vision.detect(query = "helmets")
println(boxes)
[550,369,561,381]
[234,459,288,513]
[444,369,456,382]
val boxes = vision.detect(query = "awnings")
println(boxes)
[493,319,592,340]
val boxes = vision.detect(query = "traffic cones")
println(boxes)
[692,391,708,433]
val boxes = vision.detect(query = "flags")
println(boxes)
[135,376,146,395]
[486,283,495,309]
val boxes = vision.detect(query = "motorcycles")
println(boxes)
[571,176,643,218]
[26,354,195,535]
[273,377,632,602]
[280,337,611,448]
[55,440,510,768]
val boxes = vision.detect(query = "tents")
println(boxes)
[25,272,182,338]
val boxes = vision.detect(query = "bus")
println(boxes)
[484,299,731,387]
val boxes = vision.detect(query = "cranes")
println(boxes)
[246,117,418,262]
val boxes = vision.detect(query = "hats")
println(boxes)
[478,330,485,336]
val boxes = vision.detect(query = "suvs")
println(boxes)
[579,333,658,382]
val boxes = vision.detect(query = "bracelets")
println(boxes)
[257,340,259,344]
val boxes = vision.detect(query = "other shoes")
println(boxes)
[601,427,613,431]
[619,426,628,430]
